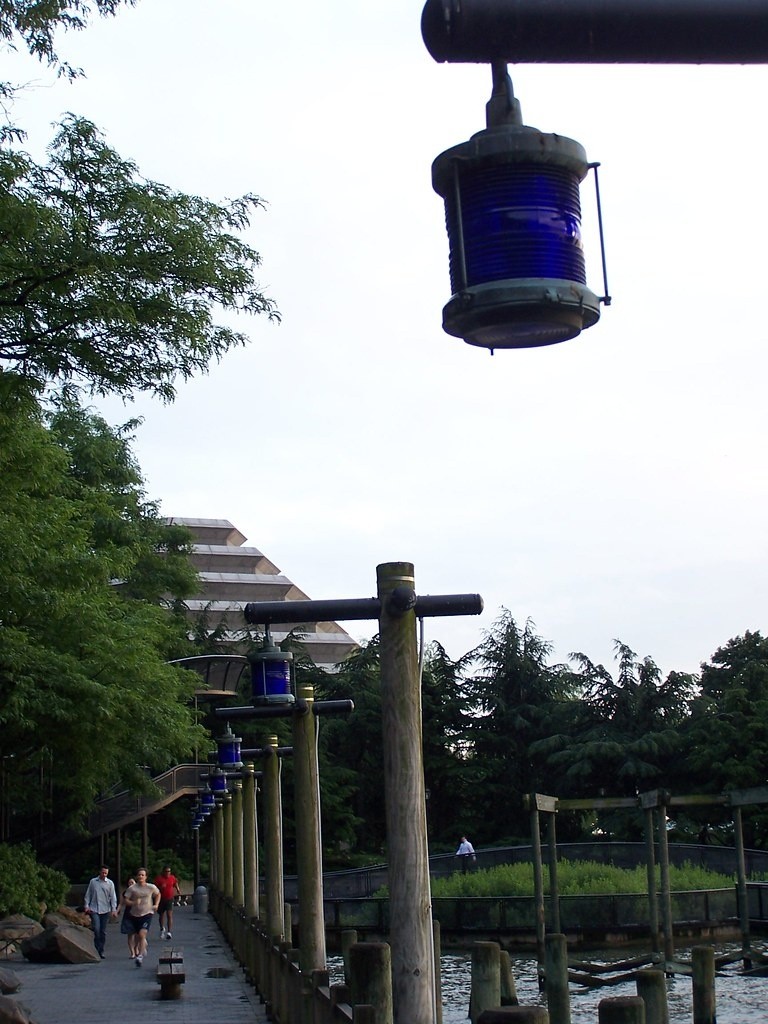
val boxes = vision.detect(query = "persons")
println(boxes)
[84,865,117,958]
[112,877,147,956]
[454,836,477,874]
[124,868,161,967]
[152,866,181,939]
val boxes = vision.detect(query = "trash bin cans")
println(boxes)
[194,885,209,915]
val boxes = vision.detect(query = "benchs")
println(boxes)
[156,964,187,999]
[159,945,185,964]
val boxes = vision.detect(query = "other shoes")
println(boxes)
[135,947,139,956]
[166,932,172,940]
[135,956,142,968]
[100,953,105,958]
[129,955,135,959]
[159,927,166,940]
